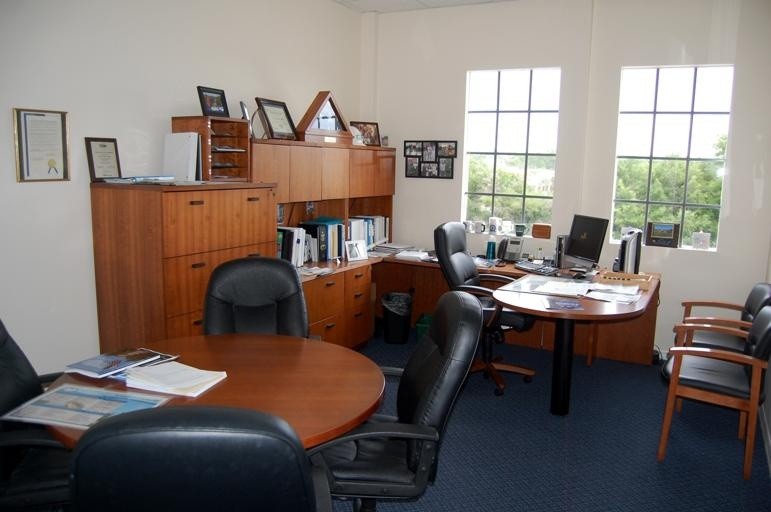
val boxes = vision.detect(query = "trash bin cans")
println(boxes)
[381,292,412,344]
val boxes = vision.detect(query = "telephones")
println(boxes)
[497,237,524,262]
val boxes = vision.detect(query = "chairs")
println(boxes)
[655,283,770,481]
[1,318,73,509]
[69,406,333,511]
[314,290,484,512]
[433,221,536,396]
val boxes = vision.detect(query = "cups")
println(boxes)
[503,221,514,233]
[488,218,502,234]
[475,222,485,233]
[463,221,474,232]
[514,224,530,236]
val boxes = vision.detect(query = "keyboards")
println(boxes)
[515,260,559,276]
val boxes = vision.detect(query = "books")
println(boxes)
[104,176,176,185]
[107,348,180,381]
[63,348,160,379]
[277,215,389,270]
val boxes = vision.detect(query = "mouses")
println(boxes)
[573,272,585,279]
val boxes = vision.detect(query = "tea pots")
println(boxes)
[619,226,642,275]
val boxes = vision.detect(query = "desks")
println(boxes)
[42,335,385,451]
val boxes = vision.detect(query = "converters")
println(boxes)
[653,349,659,362]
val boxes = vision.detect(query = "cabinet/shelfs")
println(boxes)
[239,100,250,121]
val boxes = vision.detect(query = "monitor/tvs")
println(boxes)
[565,214,609,276]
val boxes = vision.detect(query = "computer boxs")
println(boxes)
[555,234,577,268]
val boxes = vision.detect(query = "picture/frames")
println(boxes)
[196,85,230,117]
[254,96,297,140]
[645,221,680,249]
[11,107,71,183]
[403,140,458,180]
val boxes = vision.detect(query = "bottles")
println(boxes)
[556,234,566,268]
[486,235,496,260]
[613,258,620,272]
